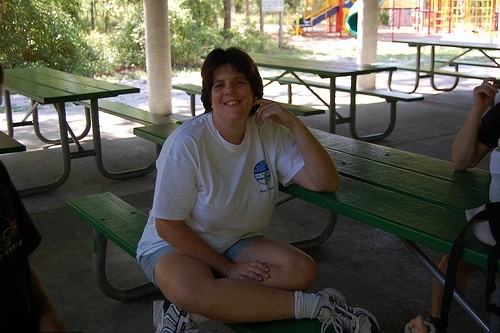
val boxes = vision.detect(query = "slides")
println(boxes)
[293,4,340,28]
[345,2,358,35]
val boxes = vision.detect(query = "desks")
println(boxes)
[202,51,397,135]
[134,121,500,333]
[392,39,500,92]
[3,66,140,181]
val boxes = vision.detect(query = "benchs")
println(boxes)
[0,60,500,333]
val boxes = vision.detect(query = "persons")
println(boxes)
[135,48,381,333]
[0,159,68,333]
[404,77,500,333]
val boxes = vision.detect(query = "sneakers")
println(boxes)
[316,288,379,333]
[152,300,199,333]
